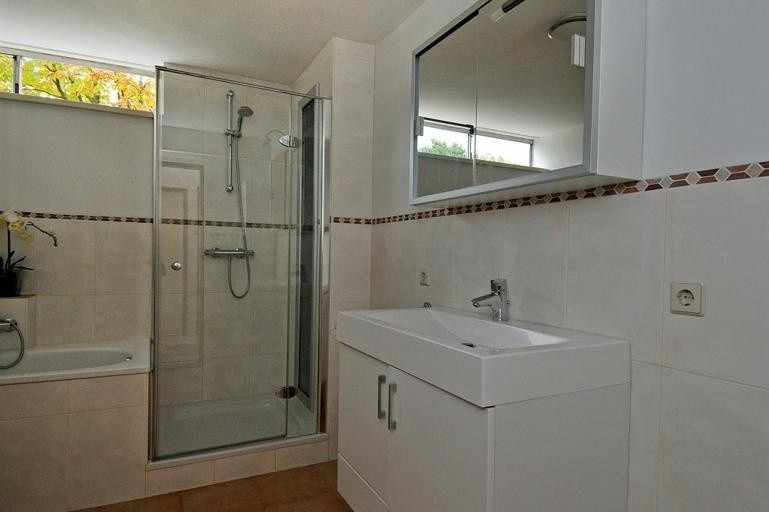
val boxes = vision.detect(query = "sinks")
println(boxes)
[332,304,629,407]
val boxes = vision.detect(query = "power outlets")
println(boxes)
[418,270,429,286]
[669,280,709,316]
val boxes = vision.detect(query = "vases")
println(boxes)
[0,271,17,298]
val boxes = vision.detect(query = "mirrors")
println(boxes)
[407,0,647,209]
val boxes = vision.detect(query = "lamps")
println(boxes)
[548,12,587,44]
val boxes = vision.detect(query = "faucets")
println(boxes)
[470,278,509,324]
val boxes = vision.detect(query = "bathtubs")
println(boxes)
[0,344,152,381]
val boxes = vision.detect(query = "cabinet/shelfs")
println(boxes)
[334,341,632,512]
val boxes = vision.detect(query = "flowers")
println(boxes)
[0,206,58,272]
